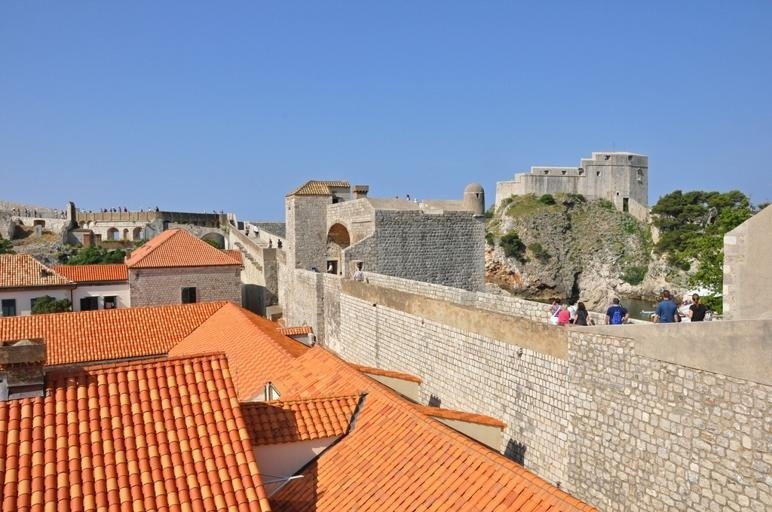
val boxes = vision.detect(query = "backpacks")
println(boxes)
[613,307,621,325]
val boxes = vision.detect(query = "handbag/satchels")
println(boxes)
[585,315,595,325]
[550,316,558,325]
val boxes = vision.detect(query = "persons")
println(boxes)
[654,290,681,323]
[550,298,592,326]
[686,293,706,321]
[605,298,629,325]
[353,266,364,282]
[13,206,159,218]
[327,262,333,274]
[407,195,410,200]
[246,225,282,249]
